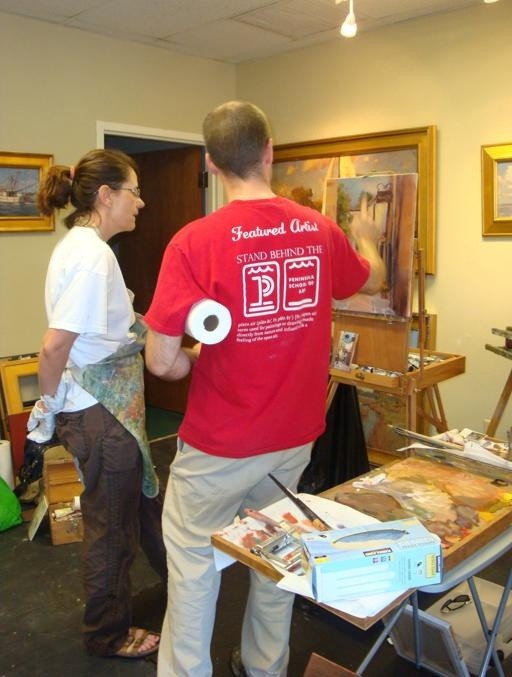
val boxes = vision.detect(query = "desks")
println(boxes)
[208,452,512,676]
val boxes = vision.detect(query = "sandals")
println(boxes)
[116,626,161,658]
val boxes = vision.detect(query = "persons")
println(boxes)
[145,101,390,675]
[28,149,168,659]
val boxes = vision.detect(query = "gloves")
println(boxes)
[40,377,67,413]
[26,400,55,443]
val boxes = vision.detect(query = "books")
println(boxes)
[334,329,359,370]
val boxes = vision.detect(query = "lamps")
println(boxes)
[340,1,360,37]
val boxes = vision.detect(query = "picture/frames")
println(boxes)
[0,151,55,234]
[384,603,473,677]
[480,141,512,237]
[271,125,440,276]
[331,331,359,371]
[1,357,41,472]
[315,174,422,319]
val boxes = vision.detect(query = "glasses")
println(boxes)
[440,594,470,613]
[91,187,141,196]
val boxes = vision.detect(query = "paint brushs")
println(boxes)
[387,422,464,451]
[268,472,331,531]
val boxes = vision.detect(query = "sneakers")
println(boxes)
[230,645,247,677]
